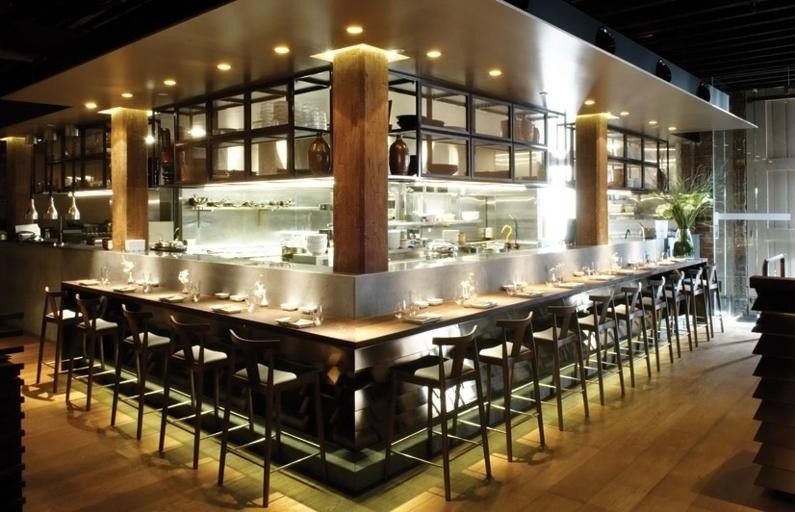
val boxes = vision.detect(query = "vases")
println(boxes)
[669,227,696,258]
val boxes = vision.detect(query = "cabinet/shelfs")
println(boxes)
[388,67,672,263]
[28,64,333,269]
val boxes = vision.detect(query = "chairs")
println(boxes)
[589,281,653,388]
[657,264,710,347]
[666,260,724,339]
[64,293,141,412]
[35,285,107,394]
[508,300,590,432]
[381,323,492,502]
[110,303,197,442]
[218,328,330,508]
[634,264,694,358]
[158,313,256,470]
[612,277,673,373]
[449,309,546,463]
[553,291,626,406]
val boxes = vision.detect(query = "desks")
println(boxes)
[58,259,717,455]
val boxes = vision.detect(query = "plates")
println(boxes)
[428,299,443,301]
[442,230,460,242]
[297,306,317,313]
[478,227,493,239]
[280,302,297,311]
[428,301,442,305]
[462,211,480,220]
[253,101,327,132]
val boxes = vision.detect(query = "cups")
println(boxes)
[101,264,111,285]
[143,273,151,293]
[190,280,200,303]
[312,304,323,327]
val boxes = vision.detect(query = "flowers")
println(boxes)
[637,163,726,253]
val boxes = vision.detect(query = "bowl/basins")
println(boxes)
[503,285,514,290]
[214,293,229,298]
[388,229,400,249]
[400,240,410,248]
[429,164,457,175]
[572,272,584,277]
[306,234,327,255]
[230,296,244,301]
[415,301,428,307]
